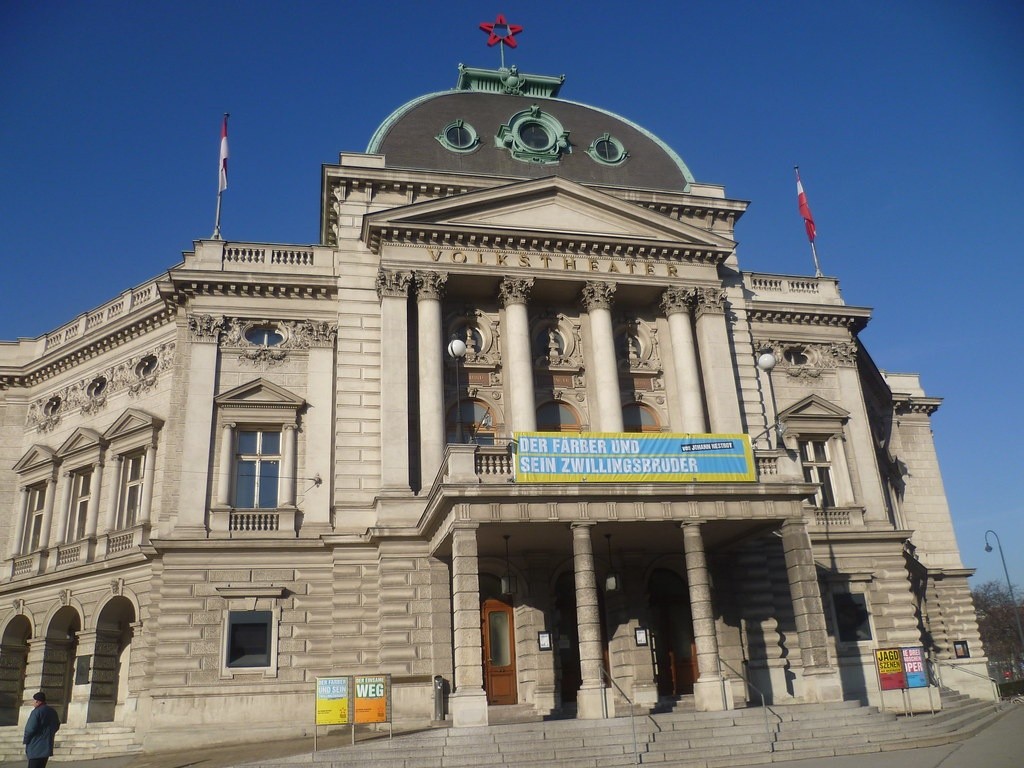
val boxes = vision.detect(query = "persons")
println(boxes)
[23,692,60,768]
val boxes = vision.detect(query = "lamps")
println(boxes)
[498,535,518,596]
[604,533,620,592]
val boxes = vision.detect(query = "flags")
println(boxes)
[219,119,230,192]
[797,175,815,242]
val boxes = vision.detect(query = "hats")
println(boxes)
[33,693,45,701]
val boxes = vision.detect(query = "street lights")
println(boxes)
[985,530,1024,646]
[757,353,794,449]
[447,332,473,444]
